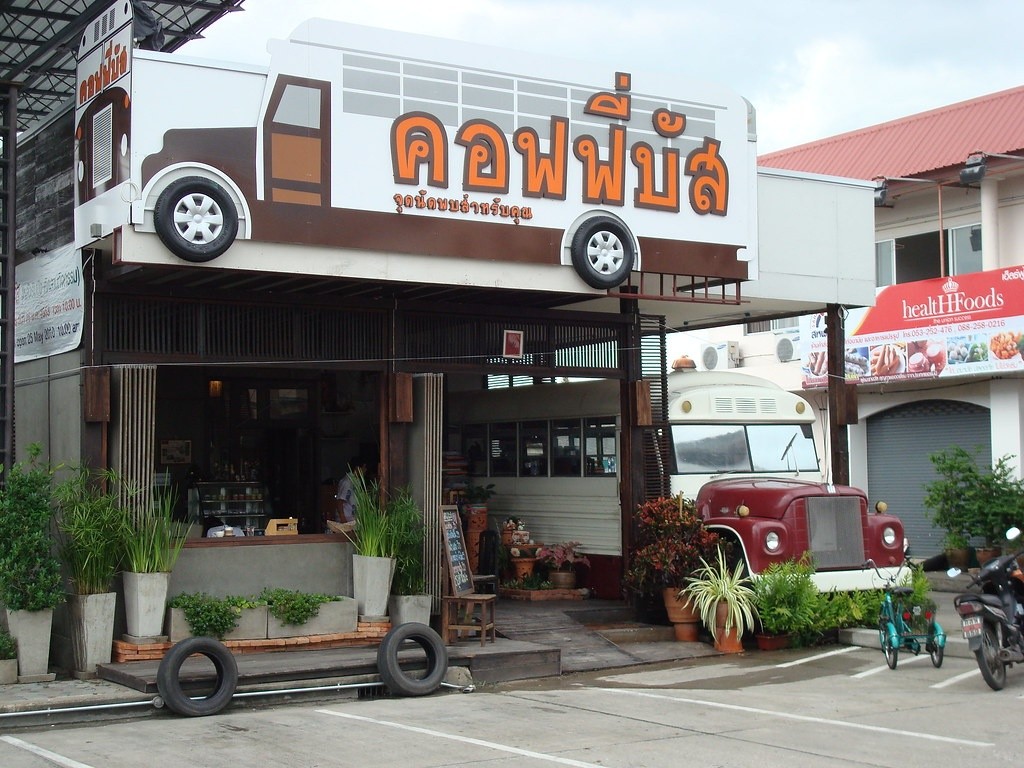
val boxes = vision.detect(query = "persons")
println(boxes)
[334,458,367,526]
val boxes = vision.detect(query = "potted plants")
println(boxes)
[678,543,763,654]
[921,444,1024,572]
[752,549,819,650]
[0,439,433,684]
[463,483,497,513]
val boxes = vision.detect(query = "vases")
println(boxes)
[503,531,512,544]
[508,545,544,583]
[664,588,700,643]
[549,573,576,589]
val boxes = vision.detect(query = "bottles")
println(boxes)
[205,459,265,517]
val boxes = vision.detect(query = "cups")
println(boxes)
[225,527,233,536]
[213,531,224,538]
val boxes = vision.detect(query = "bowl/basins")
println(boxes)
[809,362,828,377]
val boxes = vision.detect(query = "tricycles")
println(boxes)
[860,555,947,670]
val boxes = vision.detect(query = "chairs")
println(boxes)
[441,540,497,647]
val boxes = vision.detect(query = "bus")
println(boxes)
[462,354,915,605]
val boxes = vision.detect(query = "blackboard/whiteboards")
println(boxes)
[440,504,476,597]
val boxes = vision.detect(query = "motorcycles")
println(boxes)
[948,527,1024,691]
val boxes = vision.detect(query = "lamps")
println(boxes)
[960,150,1024,184]
[874,176,939,206]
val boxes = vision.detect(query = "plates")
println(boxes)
[869,344,906,373]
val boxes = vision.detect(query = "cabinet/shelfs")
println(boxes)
[187,481,266,538]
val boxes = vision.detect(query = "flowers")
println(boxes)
[624,492,734,601]
[502,513,591,572]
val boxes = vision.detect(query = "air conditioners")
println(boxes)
[699,340,739,370]
[773,333,801,365]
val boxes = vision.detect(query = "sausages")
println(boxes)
[809,350,827,376]
[871,345,902,377]
[846,348,868,375]
